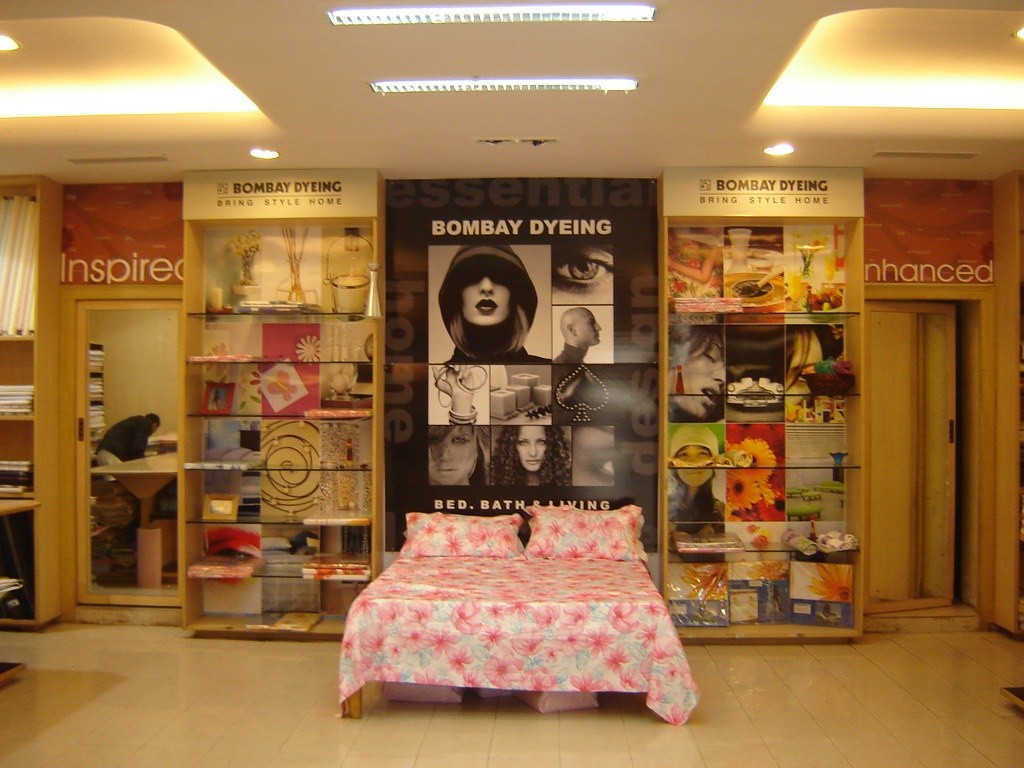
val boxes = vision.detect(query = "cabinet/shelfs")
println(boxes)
[176,166,386,643]
[0,171,62,629]
[657,167,865,639]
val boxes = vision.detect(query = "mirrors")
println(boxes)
[867,308,953,607]
[84,308,181,598]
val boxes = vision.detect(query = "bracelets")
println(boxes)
[461,366,488,390]
[438,379,454,408]
[448,403,478,425]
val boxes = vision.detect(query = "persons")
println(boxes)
[490,426,572,487]
[668,239,723,283]
[95,413,160,481]
[553,245,613,303]
[786,324,837,406]
[431,366,478,425]
[438,244,552,364]
[668,325,726,423]
[429,425,491,486]
[552,308,602,364]
[668,424,726,543]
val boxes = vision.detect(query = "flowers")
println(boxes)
[228,228,265,285]
[726,439,777,513]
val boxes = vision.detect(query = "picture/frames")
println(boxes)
[202,381,236,415]
[201,493,239,522]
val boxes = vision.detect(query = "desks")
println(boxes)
[90,452,177,528]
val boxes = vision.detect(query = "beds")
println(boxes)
[335,555,702,726]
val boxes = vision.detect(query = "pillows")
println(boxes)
[399,512,527,563]
[523,504,649,562]
